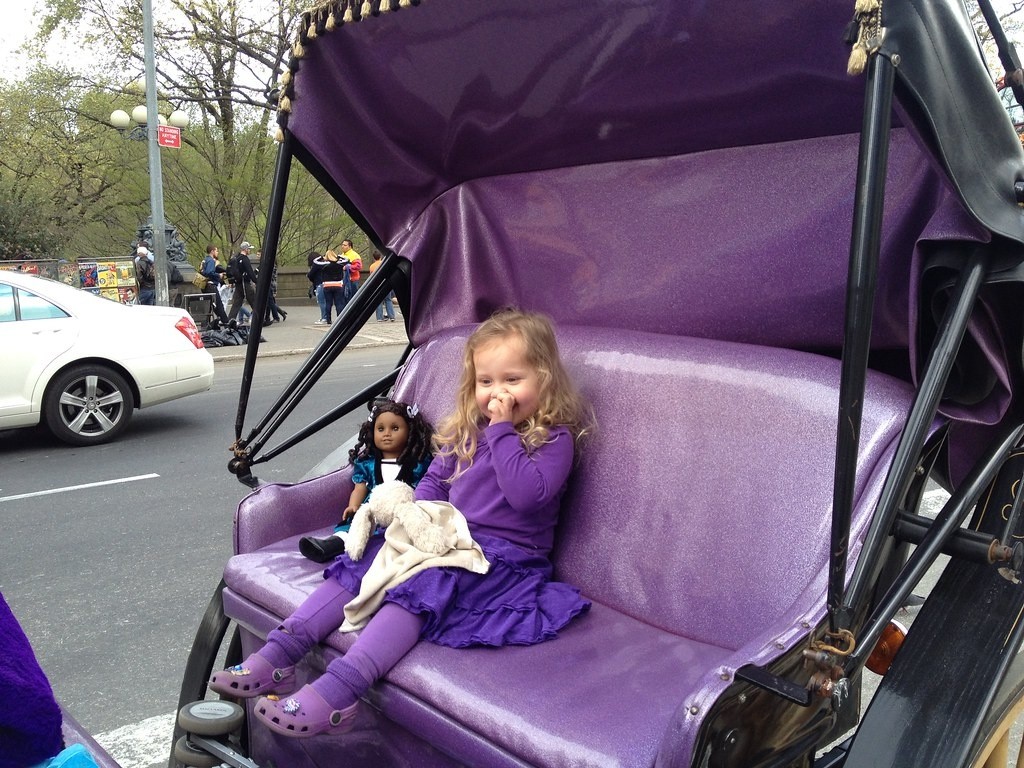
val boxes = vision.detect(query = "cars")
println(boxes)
[0,266,215,447]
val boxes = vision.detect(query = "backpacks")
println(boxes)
[167,261,183,283]
[139,259,156,281]
[226,255,245,283]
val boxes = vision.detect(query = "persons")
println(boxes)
[209,311,599,739]
[313,249,350,327]
[134,241,156,305]
[369,250,396,322]
[0,262,141,307]
[341,239,362,302]
[165,253,185,307]
[253,249,287,325]
[299,403,438,565]
[228,242,257,320]
[123,287,139,305]
[306,252,329,324]
[200,245,231,330]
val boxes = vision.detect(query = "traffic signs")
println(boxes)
[158,124,182,149]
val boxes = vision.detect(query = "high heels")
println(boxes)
[281,311,287,322]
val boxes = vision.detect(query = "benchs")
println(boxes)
[222,321,951,768]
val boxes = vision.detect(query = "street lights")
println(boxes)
[110,0,189,308]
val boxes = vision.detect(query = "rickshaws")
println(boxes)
[0,0,1019,768]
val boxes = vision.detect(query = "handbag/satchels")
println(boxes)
[192,272,209,289]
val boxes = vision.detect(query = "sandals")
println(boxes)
[208,653,297,697]
[255,683,359,736]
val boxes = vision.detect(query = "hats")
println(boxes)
[241,242,255,251]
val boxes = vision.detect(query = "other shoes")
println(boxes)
[315,319,326,325]
[391,317,396,320]
[378,317,389,323]
[271,319,279,323]
